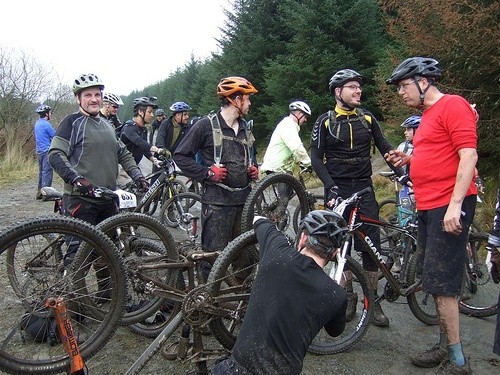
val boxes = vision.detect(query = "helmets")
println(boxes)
[289,101,311,116]
[133,97,158,109]
[217,76,258,98]
[72,73,105,94]
[299,210,348,255]
[102,93,125,106]
[385,57,443,85]
[155,109,164,116]
[329,68,369,96]
[170,102,192,111]
[400,116,422,128]
[36,104,52,114]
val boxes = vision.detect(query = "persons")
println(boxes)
[34,104,57,200]
[382,54,479,375]
[390,115,426,240]
[210,209,351,375]
[260,101,318,232]
[50,72,151,310]
[169,76,264,332]
[99,92,124,141]
[310,68,416,327]
[148,100,193,200]
[484,187,500,358]
[147,109,167,146]
[117,96,164,226]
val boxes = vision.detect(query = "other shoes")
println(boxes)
[36,192,61,202]
[95,290,132,302]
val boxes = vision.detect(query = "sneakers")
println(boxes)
[410,343,448,367]
[363,295,389,327]
[345,292,358,323]
[425,356,472,375]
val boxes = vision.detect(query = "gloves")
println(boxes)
[157,148,171,161]
[157,161,164,169]
[207,164,228,183]
[246,165,258,181]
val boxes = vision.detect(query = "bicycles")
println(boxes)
[0,149,500,375]
